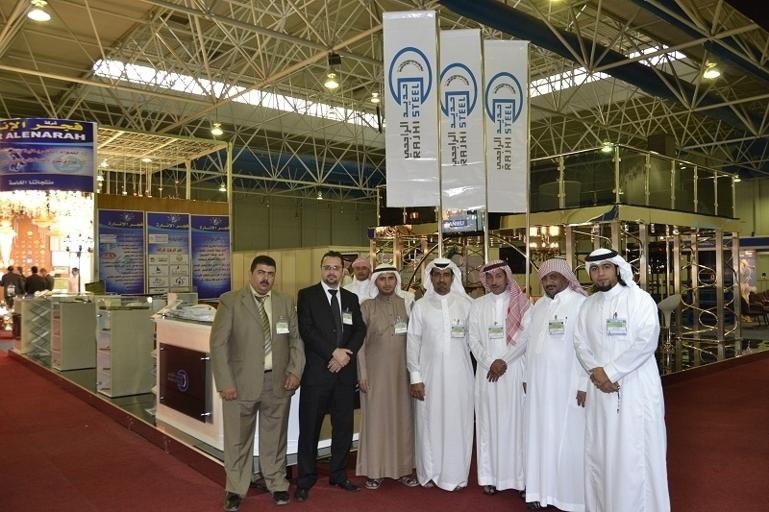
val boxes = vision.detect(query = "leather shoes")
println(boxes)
[330,479,361,491]
[295,487,308,501]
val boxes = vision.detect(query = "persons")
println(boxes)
[357,262,419,490]
[574,248,673,512]
[406,257,477,492]
[211,255,307,511]
[296,252,366,502]
[344,259,372,302]
[522,258,593,512]
[468,261,528,501]
[0,263,85,310]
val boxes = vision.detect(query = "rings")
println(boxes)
[291,384,293,386]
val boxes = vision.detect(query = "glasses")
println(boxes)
[325,265,340,270]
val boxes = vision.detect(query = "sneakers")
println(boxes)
[364,479,384,489]
[273,491,289,505]
[224,492,241,512]
[398,475,420,487]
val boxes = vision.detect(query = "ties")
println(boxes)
[328,290,342,345]
[255,296,272,355]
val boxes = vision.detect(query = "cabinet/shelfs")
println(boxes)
[97,307,155,398]
[51,302,95,371]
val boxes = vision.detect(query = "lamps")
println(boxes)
[601,136,615,155]
[323,57,339,95]
[317,185,322,200]
[219,173,227,192]
[732,172,742,184]
[210,103,225,139]
[704,41,721,81]
[26,0,51,21]
[369,79,379,106]
